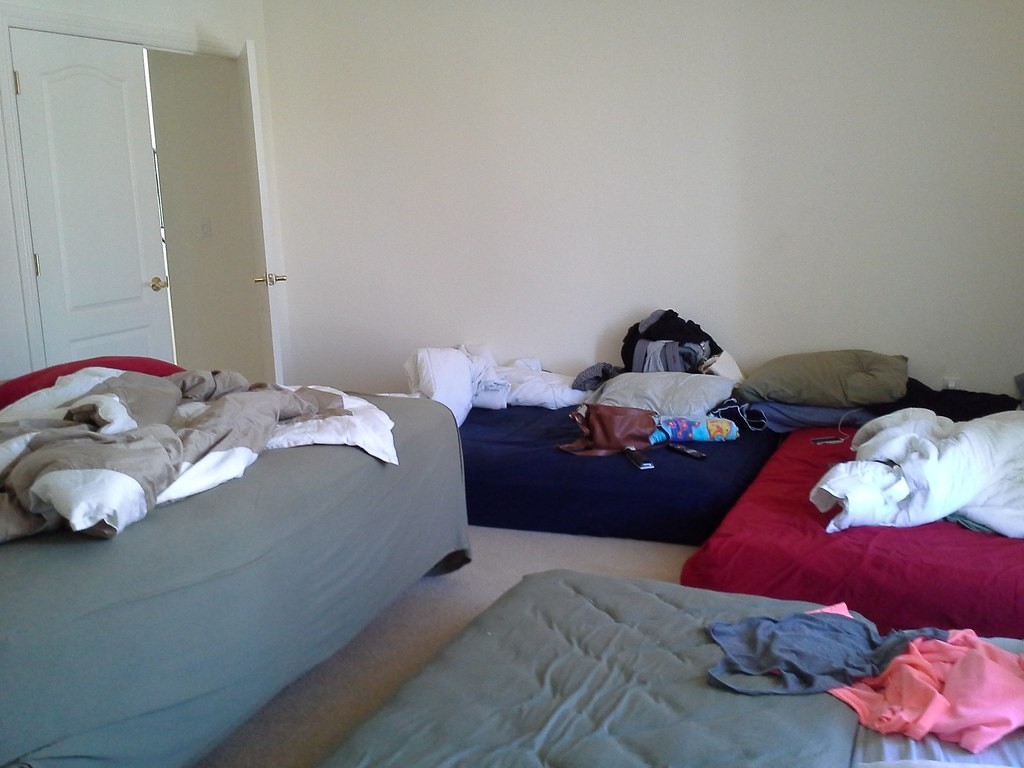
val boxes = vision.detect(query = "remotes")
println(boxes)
[669,443,707,460]
[624,445,654,469]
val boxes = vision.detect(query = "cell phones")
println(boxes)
[811,436,844,445]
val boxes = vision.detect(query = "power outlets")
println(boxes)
[942,376,961,390]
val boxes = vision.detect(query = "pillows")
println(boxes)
[741,349,909,403]
[582,370,739,419]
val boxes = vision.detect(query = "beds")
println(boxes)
[319,568,1024,768]
[460,403,777,548]
[0,357,474,768]
[689,426,1024,637]
